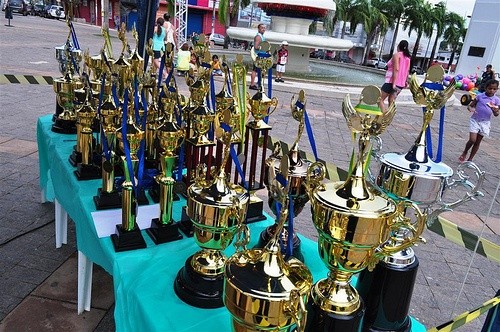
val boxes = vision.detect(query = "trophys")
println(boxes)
[52,11,278,253]
[301,85,428,332]
[173,129,250,309]
[222,178,313,332]
[253,90,326,263]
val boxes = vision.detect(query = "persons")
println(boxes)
[249,24,267,89]
[459,79,500,161]
[211,55,222,76]
[477,64,495,93]
[177,32,211,76]
[163,13,179,79]
[275,41,290,82]
[151,17,169,82]
[379,40,412,113]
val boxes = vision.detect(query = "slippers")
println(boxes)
[458,155,466,163]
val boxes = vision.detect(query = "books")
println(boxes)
[355,65,486,332]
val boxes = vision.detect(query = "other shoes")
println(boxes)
[162,74,168,81]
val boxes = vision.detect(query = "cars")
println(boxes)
[411,65,425,75]
[310,50,350,62]
[368,57,386,69]
[205,32,225,46]
[2,0,66,20]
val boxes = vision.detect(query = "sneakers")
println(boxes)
[249,85,259,90]
[275,79,284,82]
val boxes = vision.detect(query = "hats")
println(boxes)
[280,41,289,46]
[486,65,492,68]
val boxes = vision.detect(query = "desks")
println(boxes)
[38,113,427,332]
